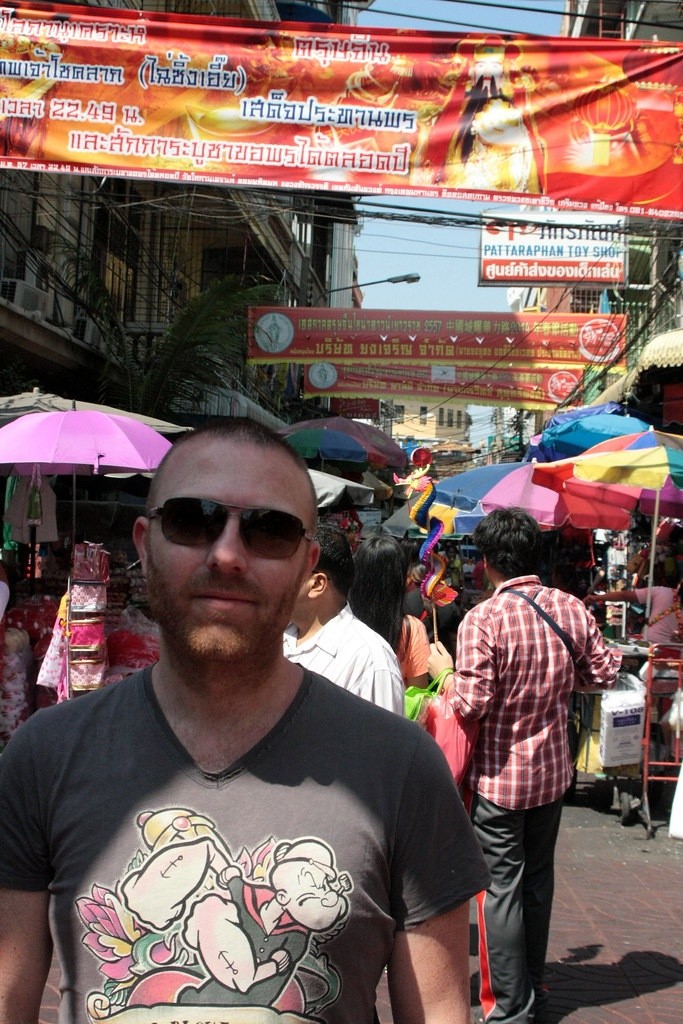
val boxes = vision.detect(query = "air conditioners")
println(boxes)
[74,317,109,352]
[0,277,50,320]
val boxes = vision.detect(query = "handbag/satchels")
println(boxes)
[405,668,479,788]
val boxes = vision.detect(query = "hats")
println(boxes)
[411,565,428,583]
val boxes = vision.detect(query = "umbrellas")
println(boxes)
[1,398,682,671]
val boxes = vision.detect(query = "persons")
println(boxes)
[350,532,428,692]
[427,506,623,1024]
[0,417,471,1024]
[583,580,683,643]
[283,524,406,718]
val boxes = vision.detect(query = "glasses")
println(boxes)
[147,497,318,559]
